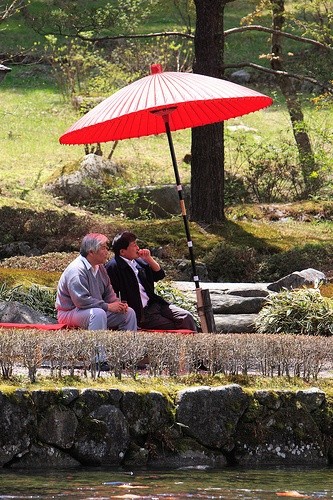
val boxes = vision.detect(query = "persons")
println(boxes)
[55,233,137,332]
[105,230,196,330]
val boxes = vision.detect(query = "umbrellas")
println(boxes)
[59,64,273,289]
